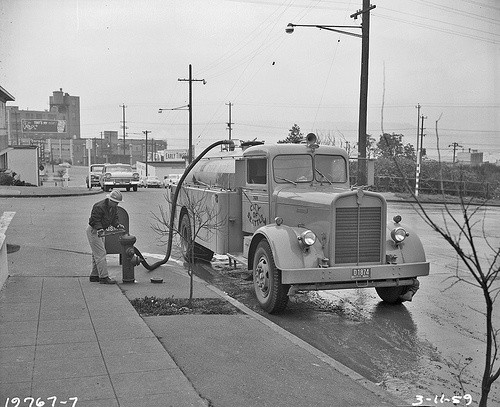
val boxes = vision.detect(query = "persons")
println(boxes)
[86,190,122,283]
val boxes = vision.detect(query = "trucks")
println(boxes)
[170,132,429,314]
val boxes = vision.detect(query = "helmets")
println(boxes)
[107,189,124,202]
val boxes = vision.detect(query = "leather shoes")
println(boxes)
[90,275,100,282]
[99,276,117,284]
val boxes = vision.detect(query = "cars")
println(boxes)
[86,164,102,189]
[99,163,139,191]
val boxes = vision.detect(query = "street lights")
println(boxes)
[159,107,191,174]
[286,22,369,187]
[177,78,207,174]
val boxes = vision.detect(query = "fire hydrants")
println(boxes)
[119,235,141,283]
